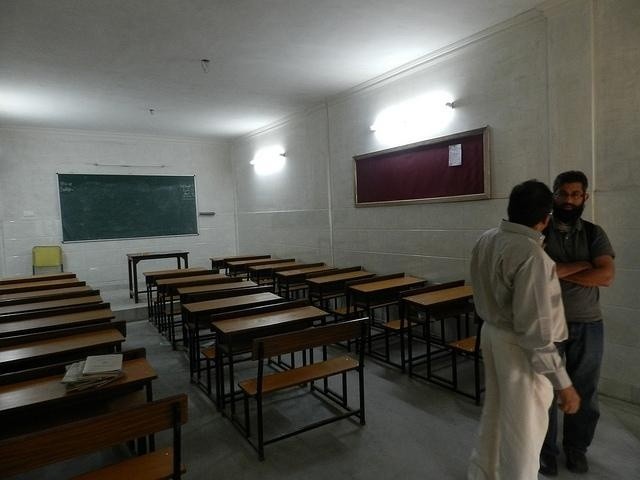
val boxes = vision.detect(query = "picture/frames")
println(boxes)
[351,124,494,207]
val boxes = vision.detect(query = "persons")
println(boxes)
[539,170,615,477]
[468,178,581,480]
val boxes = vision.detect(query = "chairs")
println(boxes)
[33,240,67,276]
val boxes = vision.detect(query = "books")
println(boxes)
[61,354,126,393]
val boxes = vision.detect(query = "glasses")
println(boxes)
[553,190,586,201]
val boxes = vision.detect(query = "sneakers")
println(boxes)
[538,450,560,476]
[562,447,590,475]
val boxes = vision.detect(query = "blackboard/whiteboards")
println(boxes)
[56,172,200,244]
